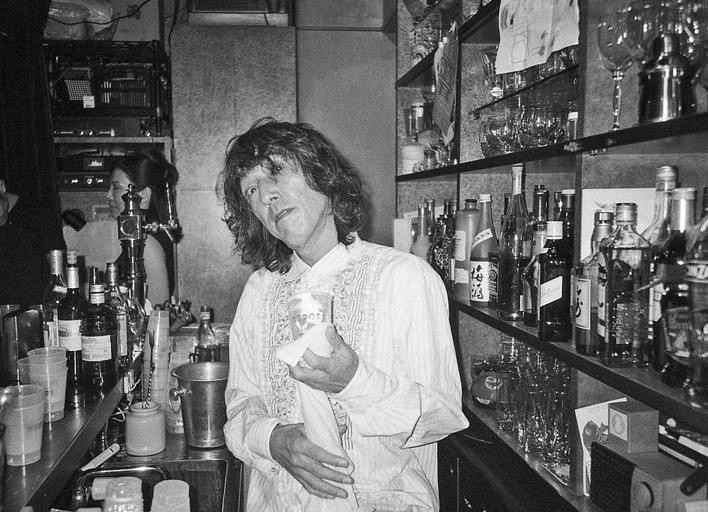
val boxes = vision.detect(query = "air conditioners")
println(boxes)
[188,0,292,28]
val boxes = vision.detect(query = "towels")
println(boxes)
[276,321,359,512]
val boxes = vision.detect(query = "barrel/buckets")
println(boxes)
[168,362,229,448]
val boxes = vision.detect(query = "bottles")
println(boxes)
[453,335,576,483]
[402,0,458,171]
[41,248,134,398]
[407,161,573,343]
[579,164,708,408]
[190,303,219,362]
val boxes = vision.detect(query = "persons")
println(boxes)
[0,147,66,305]
[108,152,179,308]
[220,122,469,512]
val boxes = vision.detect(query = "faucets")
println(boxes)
[145,186,181,242]
[71,466,170,510]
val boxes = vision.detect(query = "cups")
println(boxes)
[101,474,192,512]
[2,345,69,468]
[285,290,337,341]
[123,307,186,459]
[469,43,581,159]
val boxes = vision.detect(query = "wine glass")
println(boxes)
[592,0,708,134]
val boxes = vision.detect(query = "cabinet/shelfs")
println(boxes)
[38,40,174,189]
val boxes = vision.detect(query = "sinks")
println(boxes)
[73,456,228,511]
